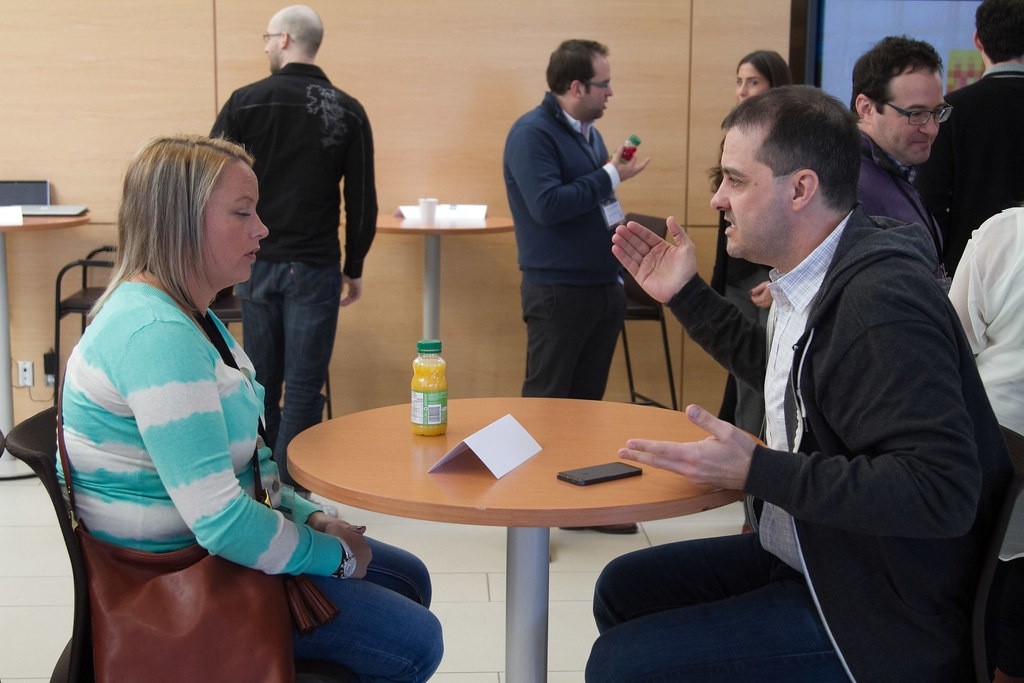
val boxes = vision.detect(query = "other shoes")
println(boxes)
[559,521,638,534]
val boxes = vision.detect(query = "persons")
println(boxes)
[56,135,444,683]
[209,5,378,518]
[707,49,801,532]
[503,39,652,534]
[946,205,1024,683]
[922,0,1024,276]
[850,37,952,274]
[586,85,1008,683]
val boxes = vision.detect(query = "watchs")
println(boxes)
[334,536,356,579]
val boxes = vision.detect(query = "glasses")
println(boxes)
[568,78,609,91]
[883,99,955,127]
[262,30,295,42]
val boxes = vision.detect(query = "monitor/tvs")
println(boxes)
[0,180,51,207]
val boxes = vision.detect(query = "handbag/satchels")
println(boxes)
[69,489,336,683]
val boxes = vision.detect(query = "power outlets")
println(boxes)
[17,361,34,387]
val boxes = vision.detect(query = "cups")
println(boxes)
[419,199,438,226]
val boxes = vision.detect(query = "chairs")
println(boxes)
[3,247,335,683]
[620,213,1024,683]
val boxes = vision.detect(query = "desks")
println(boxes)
[287,394,766,683]
[0,213,91,482]
[372,214,514,341]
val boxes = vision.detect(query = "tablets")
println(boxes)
[21,204,87,217]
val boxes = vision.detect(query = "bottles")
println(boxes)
[605,135,641,164]
[411,340,447,436]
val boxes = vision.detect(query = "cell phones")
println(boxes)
[557,462,642,486]
